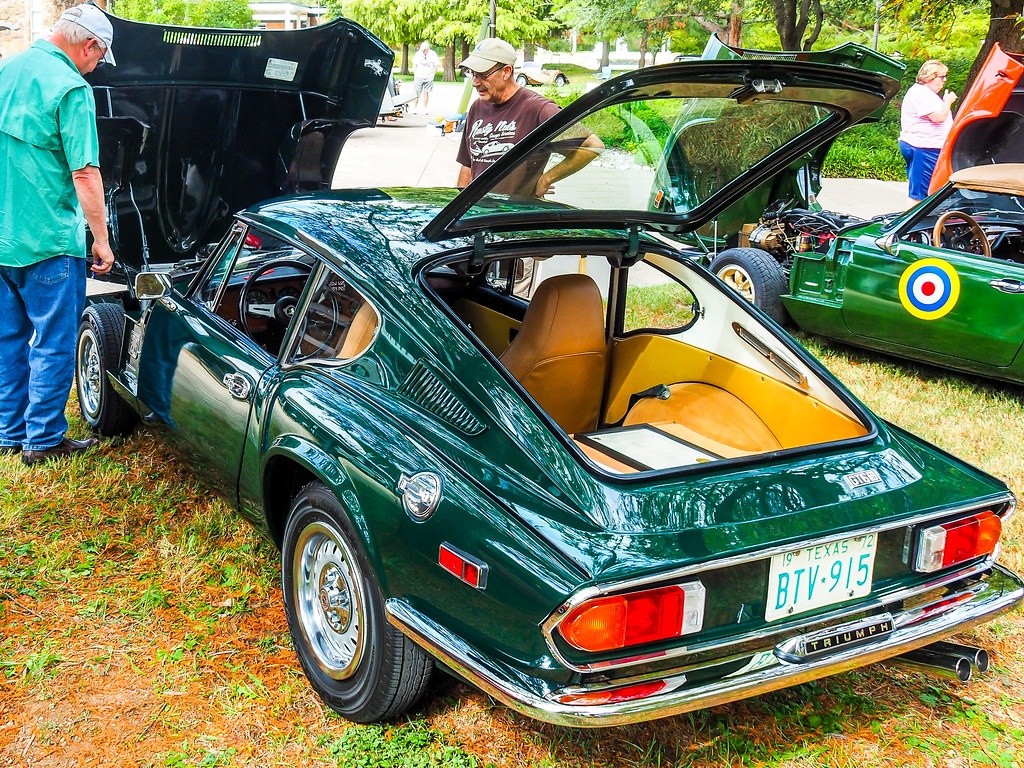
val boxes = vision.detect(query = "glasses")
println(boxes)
[933,75,947,82]
[464,66,507,79]
[87,36,108,68]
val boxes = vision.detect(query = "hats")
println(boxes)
[60,4,116,68]
[456,38,517,73]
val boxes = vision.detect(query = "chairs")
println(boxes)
[499,274,606,435]
[337,301,377,364]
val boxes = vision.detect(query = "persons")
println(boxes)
[0,4,116,465]
[898,59,957,211]
[458,38,605,301]
[412,39,441,115]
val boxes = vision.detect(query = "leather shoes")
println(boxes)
[21,437,99,466]
[3,445,22,454]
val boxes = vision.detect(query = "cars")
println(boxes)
[673,55,702,61]
[513,62,570,87]
[75,1,1024,729]
[643,31,1024,387]
[928,42,1024,223]
